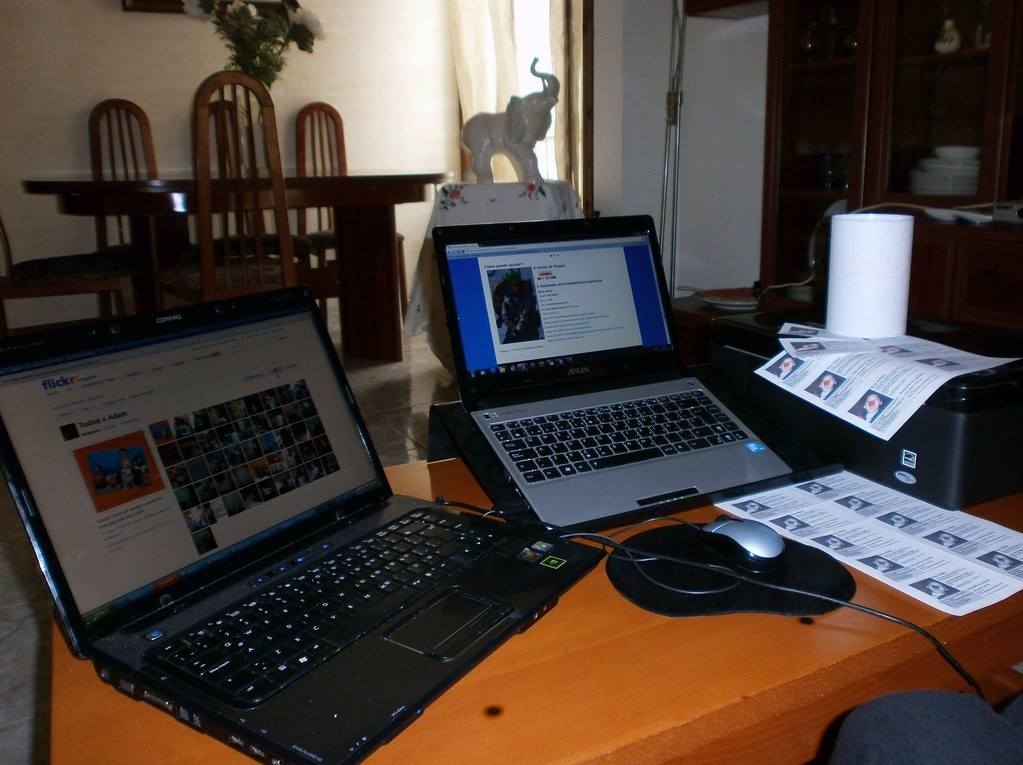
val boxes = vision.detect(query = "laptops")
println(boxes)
[0,287,606,765]
[434,215,804,535]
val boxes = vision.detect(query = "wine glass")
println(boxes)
[796,3,857,64]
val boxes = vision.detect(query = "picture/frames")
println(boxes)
[121,0,186,14]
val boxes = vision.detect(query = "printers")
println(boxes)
[709,305,1023,512]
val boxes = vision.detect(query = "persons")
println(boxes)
[809,483,825,495]
[926,581,948,599]
[992,553,1013,570]
[745,502,761,515]
[817,374,837,401]
[789,327,816,333]
[779,356,796,380]
[796,344,819,351]
[884,346,900,354]
[873,557,893,574]
[928,360,947,368]
[780,517,801,532]
[847,497,865,512]
[890,513,909,529]
[91,449,152,493]
[493,269,542,343]
[825,537,845,551]
[151,381,337,532]
[859,393,884,422]
[938,533,958,548]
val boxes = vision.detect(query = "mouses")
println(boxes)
[700,515,786,564]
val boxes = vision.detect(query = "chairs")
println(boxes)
[87,98,198,316]
[0,218,135,334]
[295,102,407,327]
[192,69,300,302]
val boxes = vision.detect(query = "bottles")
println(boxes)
[818,151,835,192]
[835,151,849,190]
[934,18,963,55]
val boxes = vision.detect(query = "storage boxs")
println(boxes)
[710,334,1023,511]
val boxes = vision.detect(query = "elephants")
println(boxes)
[459,57,560,184]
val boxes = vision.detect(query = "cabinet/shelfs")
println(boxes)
[672,0,1023,363]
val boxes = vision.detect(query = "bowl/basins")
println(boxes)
[934,145,979,159]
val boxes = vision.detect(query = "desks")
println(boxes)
[19,172,444,364]
[49,454,1023,765]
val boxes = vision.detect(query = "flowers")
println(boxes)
[183,0,329,125]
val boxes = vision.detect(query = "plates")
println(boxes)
[910,157,980,197]
[691,290,758,312]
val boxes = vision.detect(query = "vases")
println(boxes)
[239,123,270,168]
